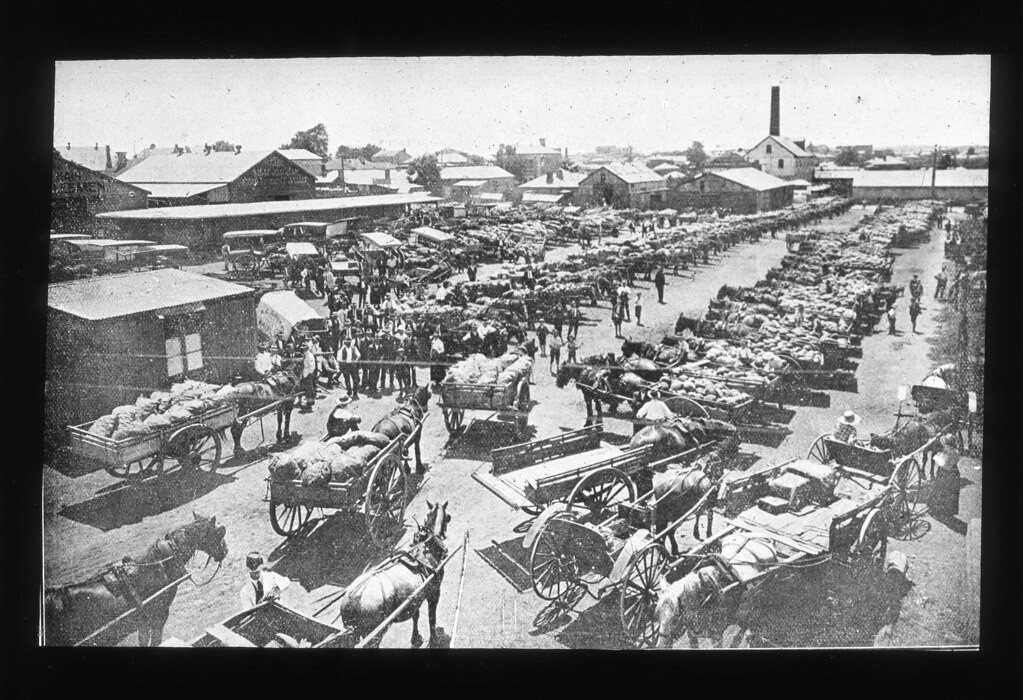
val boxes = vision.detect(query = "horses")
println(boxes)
[45,195,961,646]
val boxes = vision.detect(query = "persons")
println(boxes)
[611,218,656,237]
[627,264,635,286]
[909,273,923,307]
[909,298,920,332]
[536,282,643,372]
[887,303,897,335]
[325,393,361,436]
[834,411,861,444]
[431,330,446,382]
[934,265,948,299]
[255,208,447,414]
[598,272,609,295]
[467,260,477,282]
[240,552,291,609]
[768,215,777,239]
[436,281,455,306]
[937,196,960,240]
[788,206,822,231]
[654,269,665,304]
[637,388,674,422]
[927,432,961,515]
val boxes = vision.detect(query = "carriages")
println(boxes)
[46,191,984,648]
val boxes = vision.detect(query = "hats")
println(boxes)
[335,393,352,405]
[245,275,457,356]
